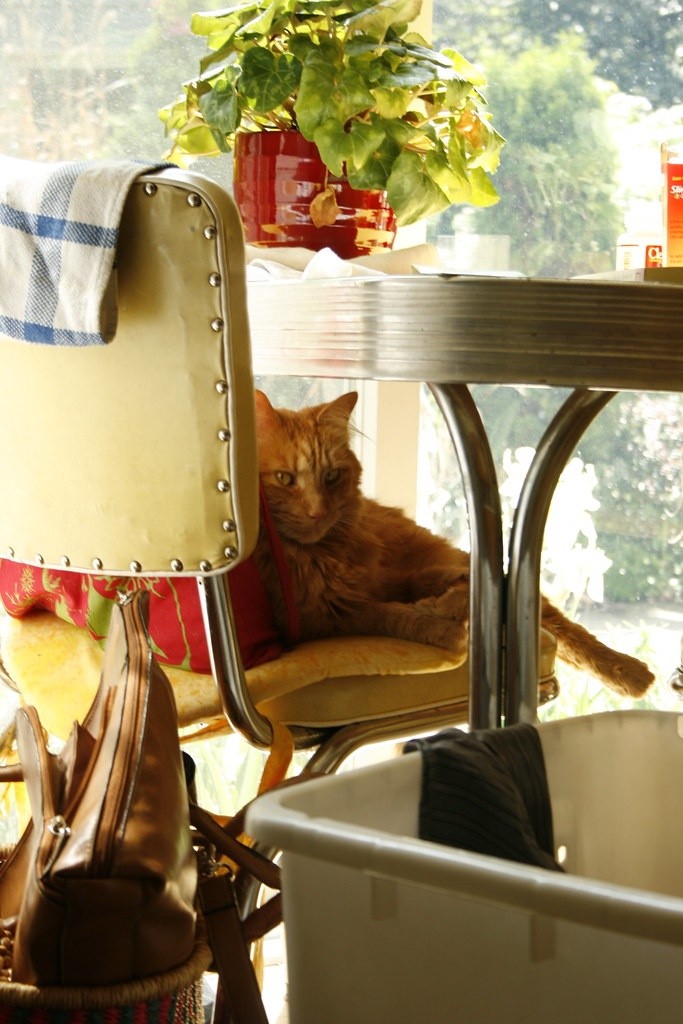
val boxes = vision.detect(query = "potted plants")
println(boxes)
[156,0,507,263]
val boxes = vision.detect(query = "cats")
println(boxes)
[254,387,654,700]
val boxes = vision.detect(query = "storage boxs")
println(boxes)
[243,706,683,1024]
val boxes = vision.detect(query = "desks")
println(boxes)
[243,273,683,730]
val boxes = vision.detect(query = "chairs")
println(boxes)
[0,149,558,935]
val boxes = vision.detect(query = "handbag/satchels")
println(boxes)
[1,586,283,1003]
